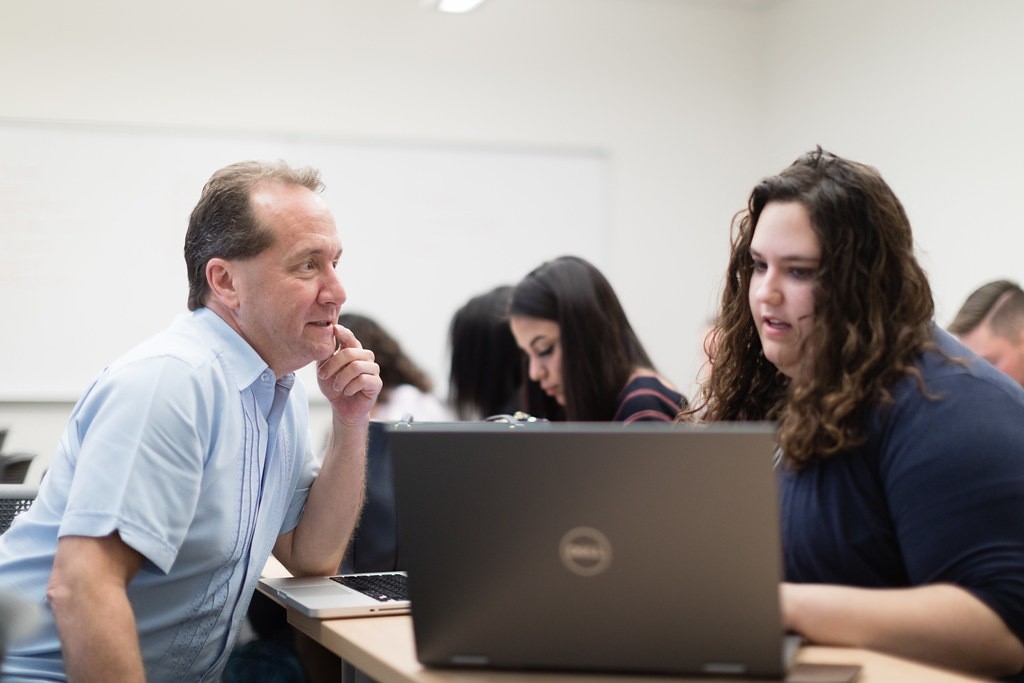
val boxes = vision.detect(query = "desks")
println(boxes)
[254,551,997,683]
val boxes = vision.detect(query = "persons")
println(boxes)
[671,145,1024,674]
[290,314,445,683]
[946,280,1024,388]
[448,286,567,422]
[0,159,379,683]
[507,256,690,421]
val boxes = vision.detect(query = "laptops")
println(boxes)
[259,572,412,618]
[386,424,802,681]
